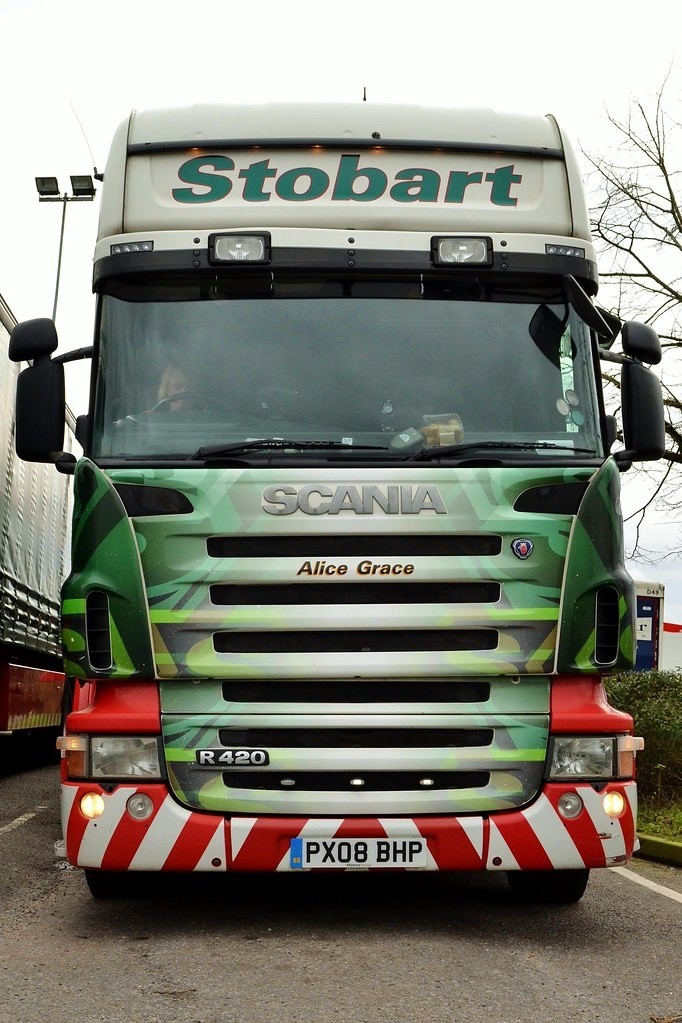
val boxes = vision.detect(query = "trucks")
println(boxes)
[2,295,87,741]
[9,100,666,911]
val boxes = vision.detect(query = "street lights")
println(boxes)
[31,175,98,323]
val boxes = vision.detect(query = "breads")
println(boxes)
[421,418,464,446]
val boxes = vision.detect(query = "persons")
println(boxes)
[142,361,198,412]
